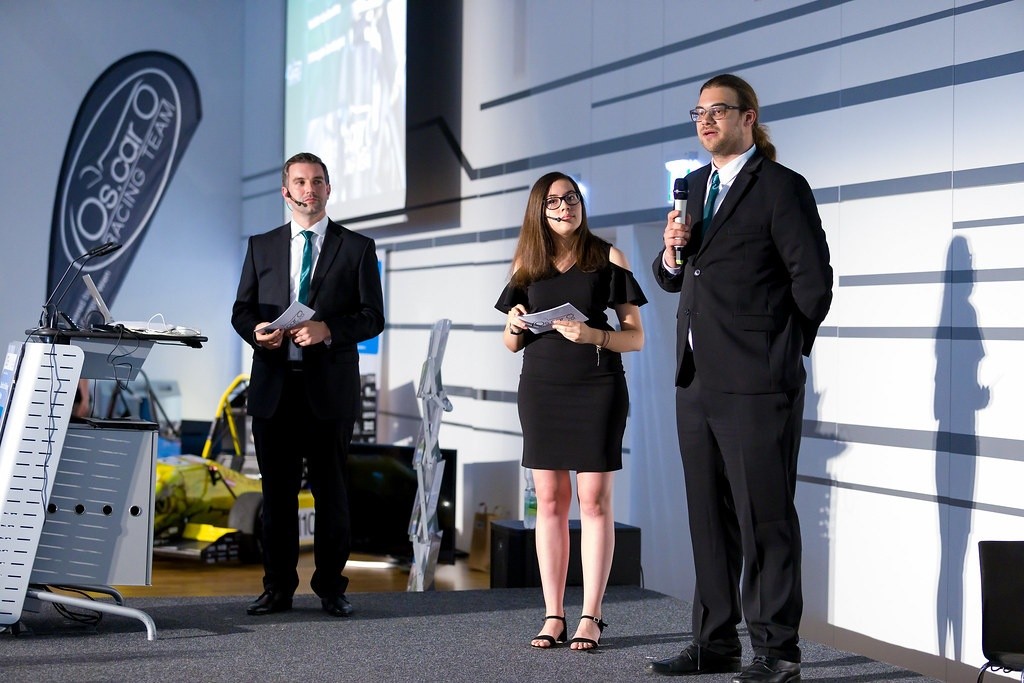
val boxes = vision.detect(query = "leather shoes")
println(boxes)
[652,644,743,675]
[731,655,801,683]
[321,593,352,616]
[247,590,293,615]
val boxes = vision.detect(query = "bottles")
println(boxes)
[523,468,537,529]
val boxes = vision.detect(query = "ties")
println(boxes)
[699,172,720,242]
[292,230,314,348]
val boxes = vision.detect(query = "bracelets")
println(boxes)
[511,331,522,335]
[596,331,610,366]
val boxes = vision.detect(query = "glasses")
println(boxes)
[690,104,748,122]
[543,192,580,210]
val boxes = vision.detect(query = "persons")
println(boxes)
[231,153,384,617]
[73,379,94,416]
[647,75,833,683]
[494,172,648,650]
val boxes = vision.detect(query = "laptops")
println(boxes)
[84,274,148,332]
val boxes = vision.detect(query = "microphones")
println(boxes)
[543,215,561,222]
[672,177,690,265]
[285,191,301,206]
[40,242,123,329]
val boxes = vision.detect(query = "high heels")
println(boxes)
[532,613,567,647]
[571,616,608,650]
